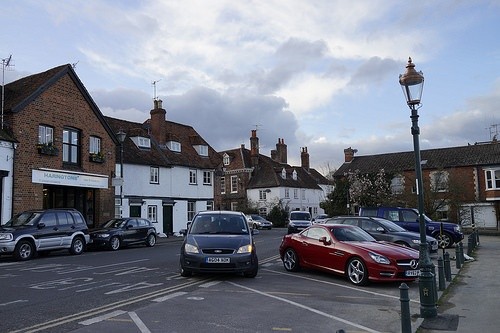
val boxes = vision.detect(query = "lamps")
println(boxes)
[141,201,147,206]
[173,202,178,206]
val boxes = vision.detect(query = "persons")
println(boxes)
[196,217,221,232]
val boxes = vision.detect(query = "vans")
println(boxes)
[287,210,313,234]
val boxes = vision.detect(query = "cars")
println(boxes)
[313,214,329,224]
[178,209,259,278]
[86,217,157,251]
[246,214,273,230]
[324,214,440,253]
[278,223,420,286]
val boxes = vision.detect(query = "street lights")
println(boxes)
[115,126,127,219]
[397,56,440,318]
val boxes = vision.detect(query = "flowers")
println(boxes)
[36,141,61,151]
[91,152,107,158]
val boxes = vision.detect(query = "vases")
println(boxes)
[38,147,59,156]
[89,157,103,162]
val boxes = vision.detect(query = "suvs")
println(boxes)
[357,206,463,249]
[0,207,90,261]
